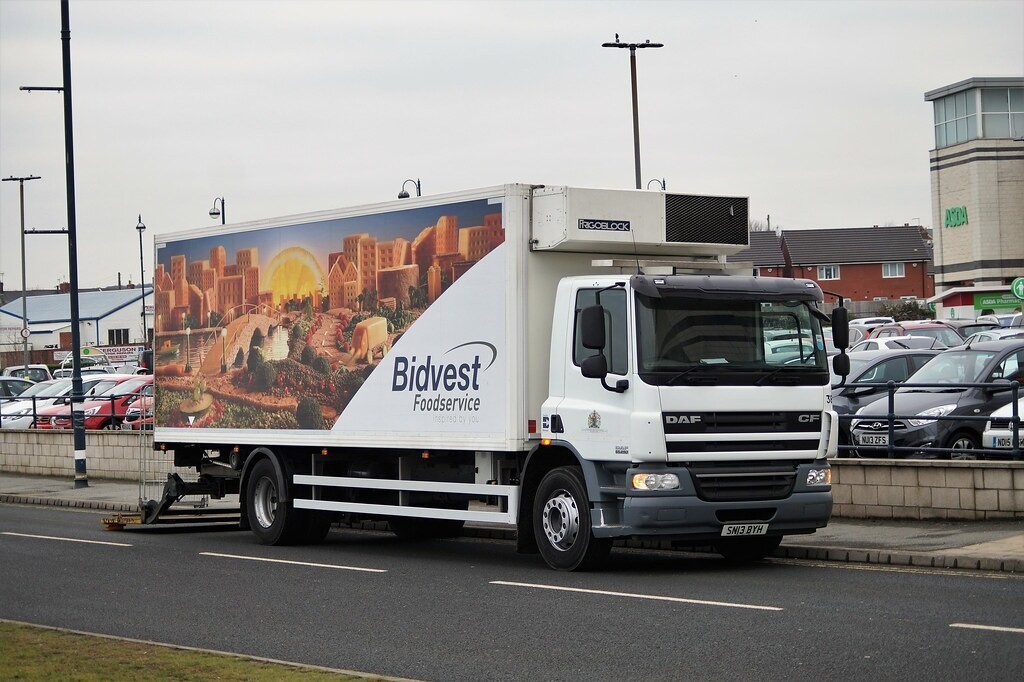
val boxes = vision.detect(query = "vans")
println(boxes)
[2,364,54,382]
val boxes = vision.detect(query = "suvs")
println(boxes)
[938,319,1003,342]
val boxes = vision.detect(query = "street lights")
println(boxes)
[398,179,421,197]
[209,196,226,225]
[136,214,147,342]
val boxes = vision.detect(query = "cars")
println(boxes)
[961,329,1024,344]
[849,338,1024,456]
[0,374,155,432]
[865,321,966,350]
[826,352,956,456]
[762,317,896,365]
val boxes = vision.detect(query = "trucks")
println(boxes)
[152,183,850,573]
